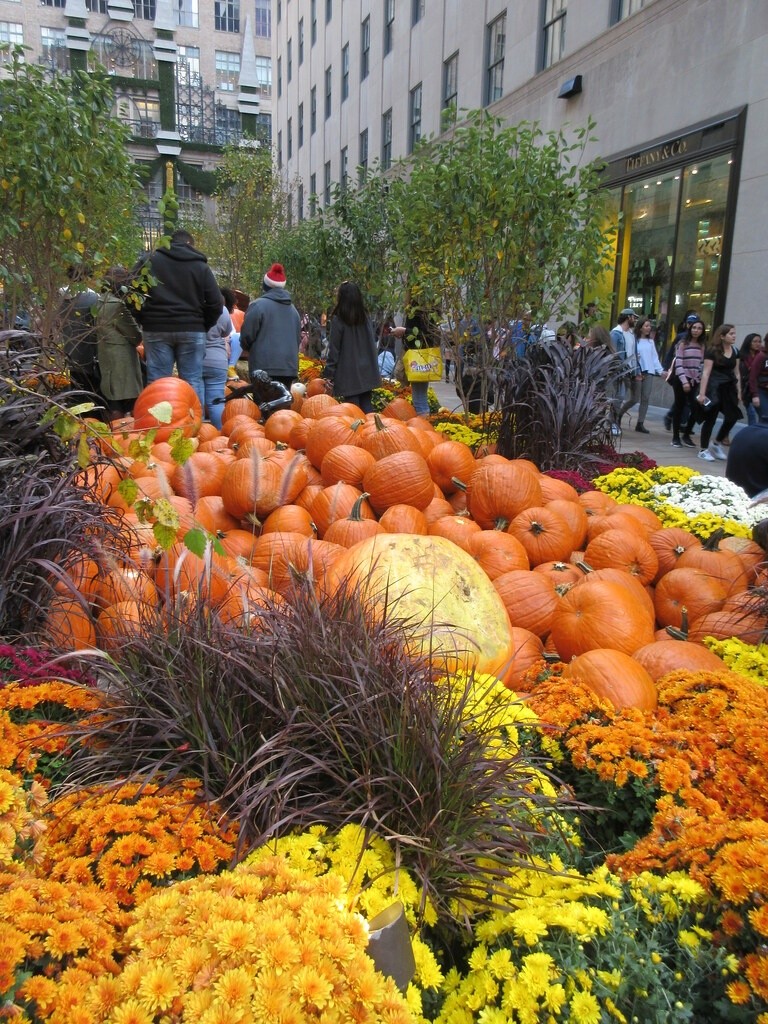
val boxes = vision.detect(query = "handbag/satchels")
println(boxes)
[402,334,443,382]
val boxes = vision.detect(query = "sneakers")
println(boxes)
[711,444,727,459]
[671,437,681,448]
[698,449,716,461]
[228,366,240,380]
[682,436,695,448]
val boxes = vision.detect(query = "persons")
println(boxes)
[48,229,767,500]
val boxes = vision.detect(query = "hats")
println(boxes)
[686,315,698,323]
[621,309,640,318]
[263,264,287,289]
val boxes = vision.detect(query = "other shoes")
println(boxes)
[636,422,649,433]
[612,424,621,435]
[664,417,671,430]
[681,427,694,434]
[722,436,730,446]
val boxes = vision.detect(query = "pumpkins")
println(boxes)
[38,376,768,716]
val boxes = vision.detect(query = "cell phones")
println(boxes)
[388,327,401,338]
[696,395,711,406]
